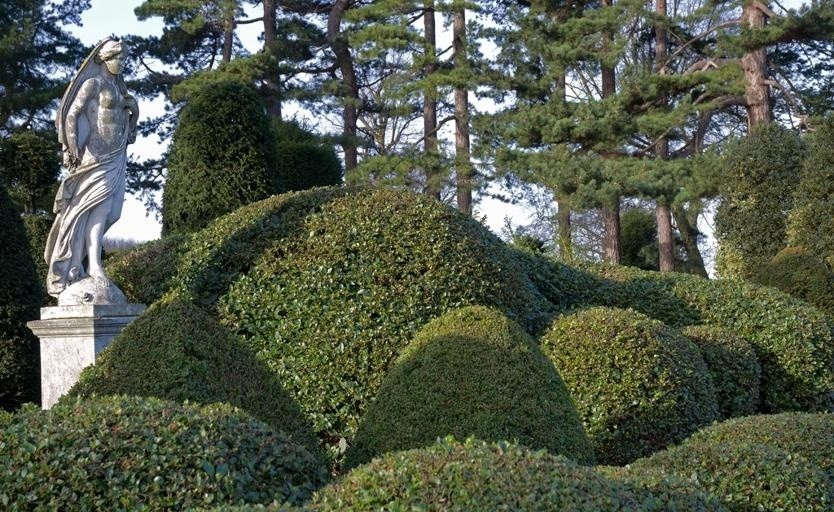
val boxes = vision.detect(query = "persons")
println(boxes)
[41,37,139,299]
